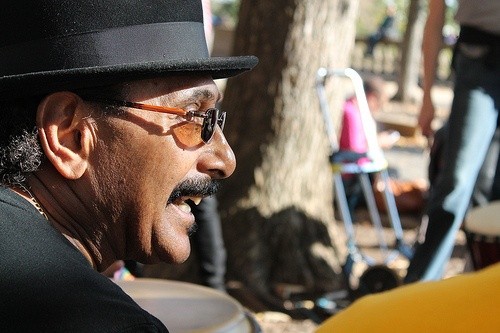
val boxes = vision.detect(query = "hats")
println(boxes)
[0,0,259,90]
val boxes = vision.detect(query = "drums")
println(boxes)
[110,276,262,333]
[460,199,500,271]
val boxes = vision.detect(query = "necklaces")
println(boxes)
[12,174,50,221]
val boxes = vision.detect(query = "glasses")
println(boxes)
[80,97,226,144]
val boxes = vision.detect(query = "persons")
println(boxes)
[363,5,398,59]
[0,0,237,333]
[333,76,393,224]
[399,0,500,286]
